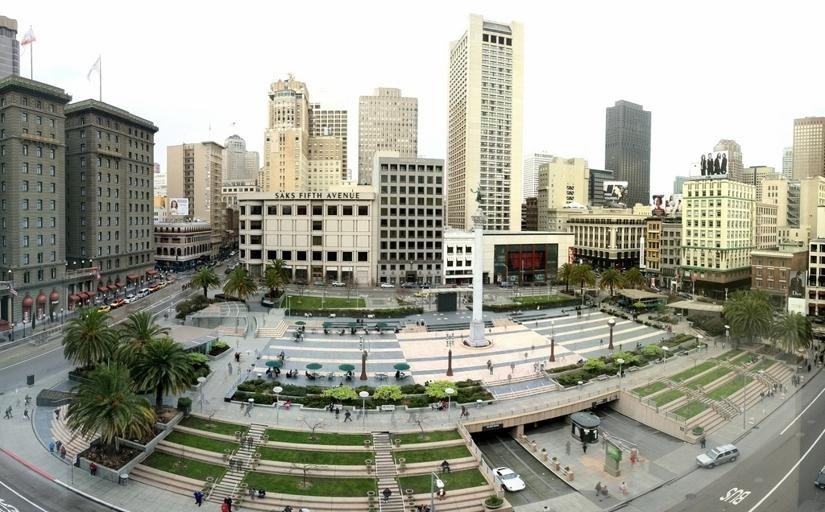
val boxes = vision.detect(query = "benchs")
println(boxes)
[328,405,343,412]
[433,402,445,411]
[381,404,395,413]
[277,400,290,409]
[598,374,609,381]
[655,357,664,364]
[628,365,638,372]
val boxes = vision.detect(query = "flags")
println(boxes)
[86,57,100,80]
[19,28,35,46]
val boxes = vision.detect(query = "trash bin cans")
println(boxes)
[248,398,254,407]
[27,376,34,385]
[120,474,128,486]
[577,380,583,390]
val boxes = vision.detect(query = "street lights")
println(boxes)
[446,332,454,376]
[549,319,556,362]
[430,471,445,512]
[359,391,369,425]
[445,387,454,424]
[196,376,206,414]
[360,336,370,380]
[743,369,765,430]
[9,307,63,341]
[607,318,731,378]
[273,386,283,425]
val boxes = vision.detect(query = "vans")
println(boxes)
[401,282,416,289]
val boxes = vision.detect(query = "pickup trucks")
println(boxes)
[419,282,431,289]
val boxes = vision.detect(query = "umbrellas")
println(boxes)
[338,363,356,371]
[306,362,323,372]
[266,357,284,368]
[376,320,386,329]
[322,321,332,328]
[393,360,411,371]
[348,321,358,327]
[294,320,307,325]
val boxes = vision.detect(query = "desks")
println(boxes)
[256,369,411,382]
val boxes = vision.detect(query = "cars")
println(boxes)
[695,443,740,469]
[332,280,346,287]
[493,467,526,493]
[380,282,396,289]
[97,276,176,313]
[814,464,825,489]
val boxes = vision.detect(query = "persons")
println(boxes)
[700,436,706,448]
[720,153,728,175]
[1,391,68,461]
[190,397,354,511]
[619,481,628,496]
[441,459,452,473]
[700,154,707,176]
[90,461,97,476]
[599,484,610,501]
[714,152,722,175]
[652,195,666,216]
[170,200,178,215]
[595,480,602,496]
[707,152,714,175]
